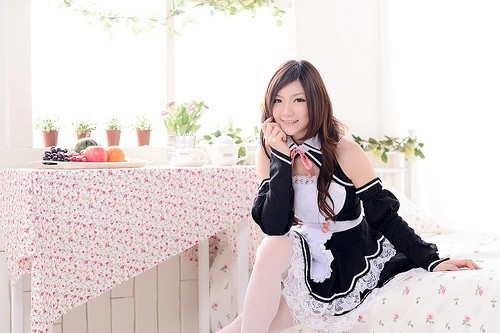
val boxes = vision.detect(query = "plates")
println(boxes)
[36,160,142,168]
[172,160,206,166]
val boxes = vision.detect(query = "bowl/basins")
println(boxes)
[205,143,238,166]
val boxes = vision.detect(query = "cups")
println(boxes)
[175,149,202,161]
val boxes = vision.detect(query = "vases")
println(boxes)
[386,152,401,164]
[175,136,199,167]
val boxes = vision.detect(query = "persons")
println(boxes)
[214,59,483,333]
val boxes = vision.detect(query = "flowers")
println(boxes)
[353,134,426,164]
[160,100,210,135]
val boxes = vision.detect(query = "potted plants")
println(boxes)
[106,118,120,147]
[198,126,246,167]
[136,120,151,147]
[244,127,261,166]
[38,119,59,147]
[73,122,96,139]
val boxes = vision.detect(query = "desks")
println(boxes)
[0,165,266,333]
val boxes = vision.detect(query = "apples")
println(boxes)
[74,138,98,153]
[84,146,108,162]
[107,146,125,162]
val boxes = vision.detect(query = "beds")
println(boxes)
[209,184,500,333]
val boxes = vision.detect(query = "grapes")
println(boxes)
[43,146,70,161]
[68,152,87,162]
